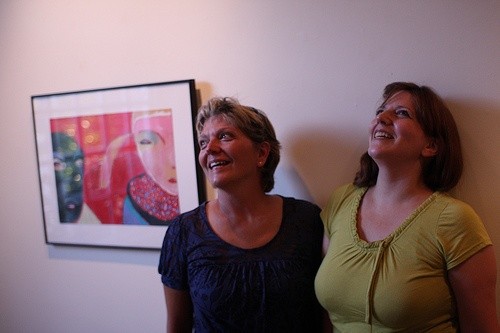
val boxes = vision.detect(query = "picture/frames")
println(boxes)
[30,78,206,250]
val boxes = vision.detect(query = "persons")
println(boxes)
[158,95,333,332]
[314,80,500,333]
